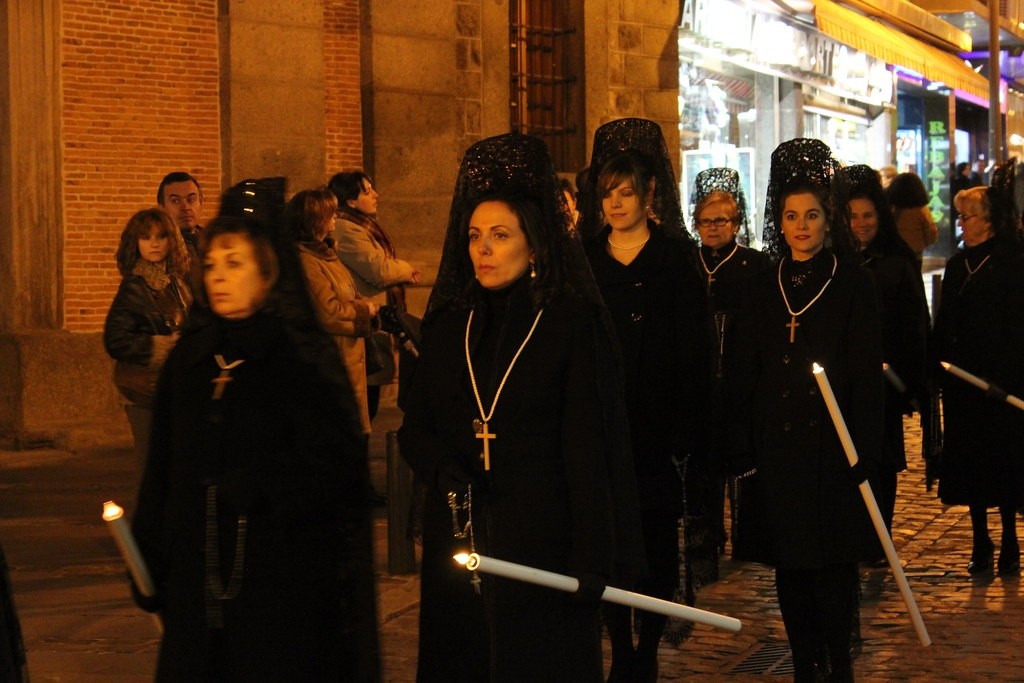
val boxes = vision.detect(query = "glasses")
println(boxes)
[697,218,735,227]
[958,214,979,224]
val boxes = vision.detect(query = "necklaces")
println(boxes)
[465,305,544,472]
[608,233,650,250]
[699,245,739,286]
[211,354,241,402]
[956,254,991,296]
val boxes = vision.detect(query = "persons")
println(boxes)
[327,168,422,514]
[1014,161,1024,230]
[282,185,376,450]
[156,171,203,262]
[103,202,194,468]
[951,159,985,219]
[575,119,939,683]
[556,177,581,236]
[779,253,838,343]
[396,130,649,683]
[126,176,386,683]
[931,185,1024,574]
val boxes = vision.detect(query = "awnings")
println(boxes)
[814,0,1004,104]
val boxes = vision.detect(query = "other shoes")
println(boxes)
[969,542,993,573]
[999,543,1019,576]
